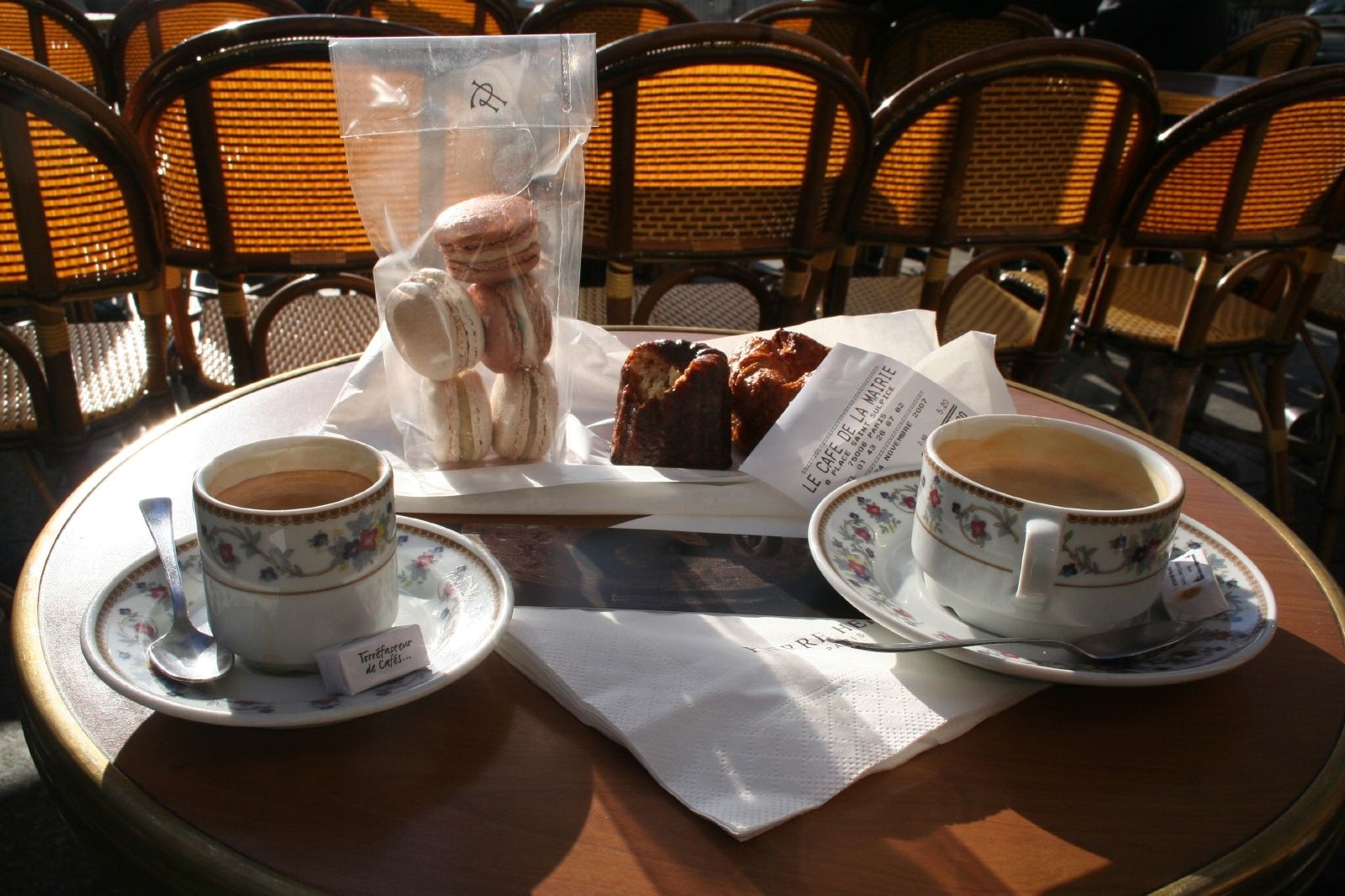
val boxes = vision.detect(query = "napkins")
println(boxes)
[491,602,1056,841]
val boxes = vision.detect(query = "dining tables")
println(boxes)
[14,326,1345,896]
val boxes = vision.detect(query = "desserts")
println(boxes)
[464,274,553,373]
[488,362,559,462]
[608,337,732,472]
[432,194,541,283]
[726,328,834,457]
[421,371,494,464]
[383,267,485,381]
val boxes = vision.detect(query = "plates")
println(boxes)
[807,465,1278,686]
[81,512,515,728]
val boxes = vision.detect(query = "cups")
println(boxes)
[190,434,400,672]
[912,414,1186,645]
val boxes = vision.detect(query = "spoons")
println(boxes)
[818,619,1209,664]
[138,495,234,683]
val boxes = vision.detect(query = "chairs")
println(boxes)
[0,0,1345,532]
